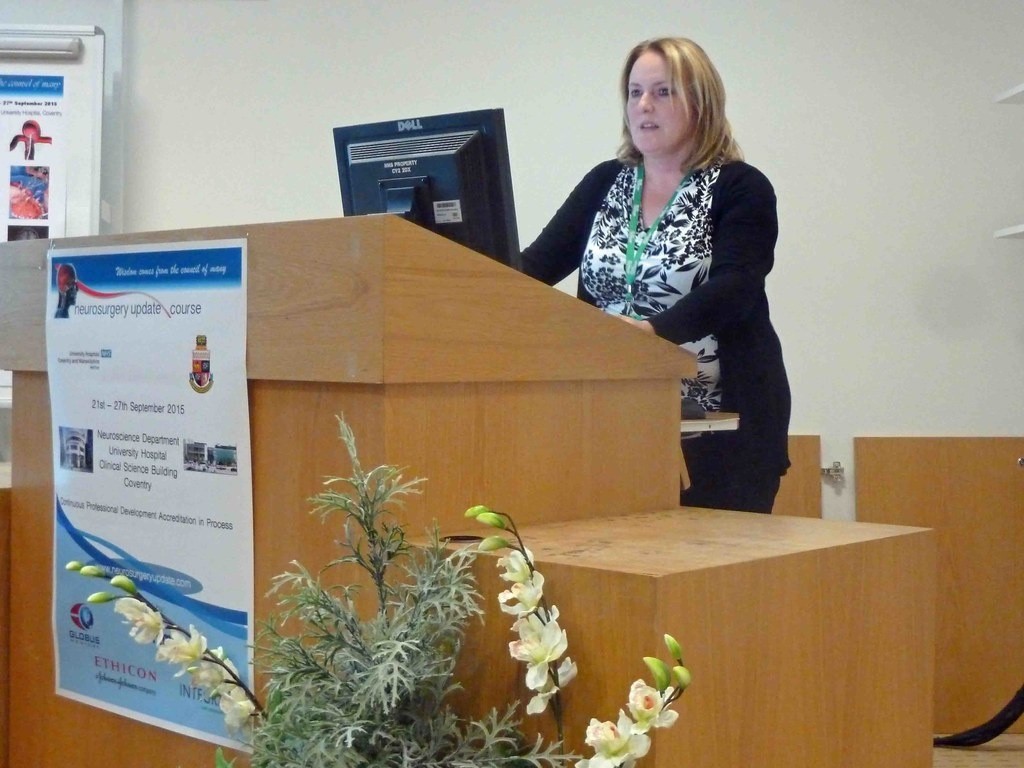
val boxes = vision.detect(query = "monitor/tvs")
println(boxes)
[331,107,520,272]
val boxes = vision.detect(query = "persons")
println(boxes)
[520,36,792,513]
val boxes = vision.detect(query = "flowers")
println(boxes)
[65,416,690,768]
